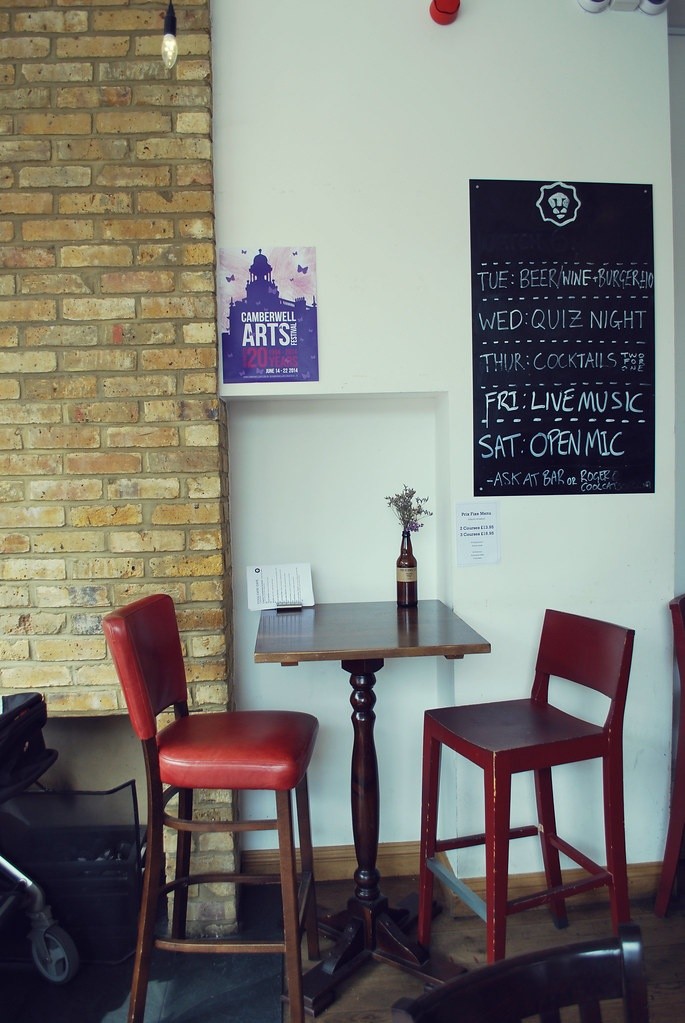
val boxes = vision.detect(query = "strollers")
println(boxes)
[0,690,81,985]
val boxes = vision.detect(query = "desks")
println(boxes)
[252,601,496,1018]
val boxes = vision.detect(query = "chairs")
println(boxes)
[653,589,685,918]
[415,606,639,965]
[393,921,653,1023]
[102,594,330,1023]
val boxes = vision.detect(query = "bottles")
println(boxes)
[396,531,417,607]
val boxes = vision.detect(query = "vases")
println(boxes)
[396,531,419,606]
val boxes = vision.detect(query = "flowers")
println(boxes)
[384,484,432,534]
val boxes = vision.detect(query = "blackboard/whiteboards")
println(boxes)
[469,179,655,497]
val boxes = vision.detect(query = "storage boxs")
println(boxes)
[9,824,148,958]
